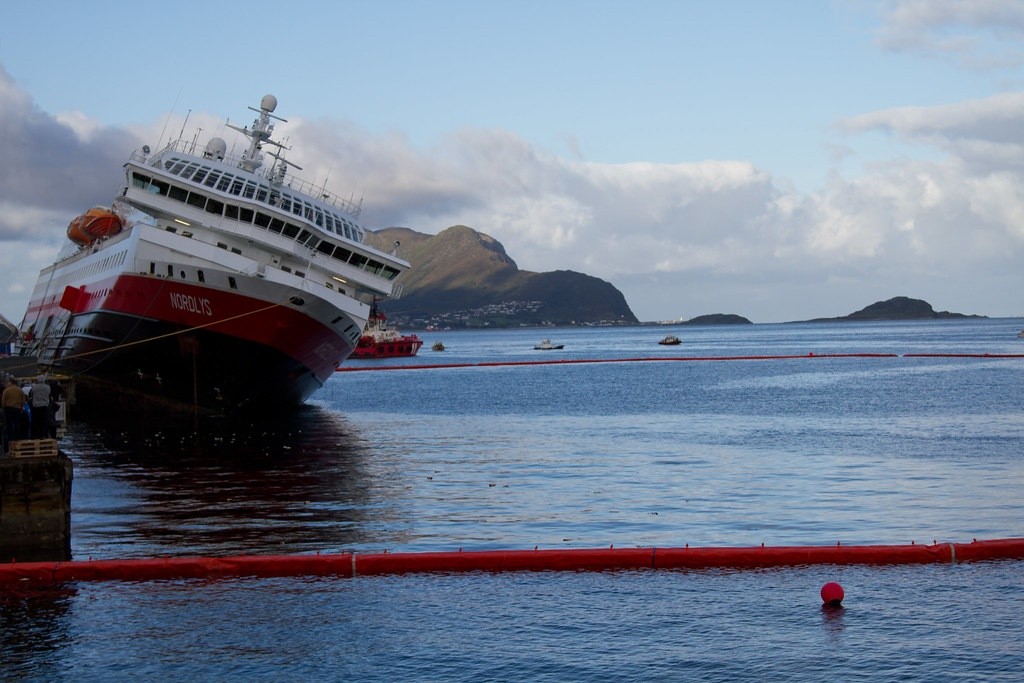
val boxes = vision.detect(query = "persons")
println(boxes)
[26,375,52,440]
[2,376,26,441]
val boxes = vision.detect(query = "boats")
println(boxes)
[533,339,564,351]
[14,93,412,414]
[657,335,681,345]
[343,297,423,359]
